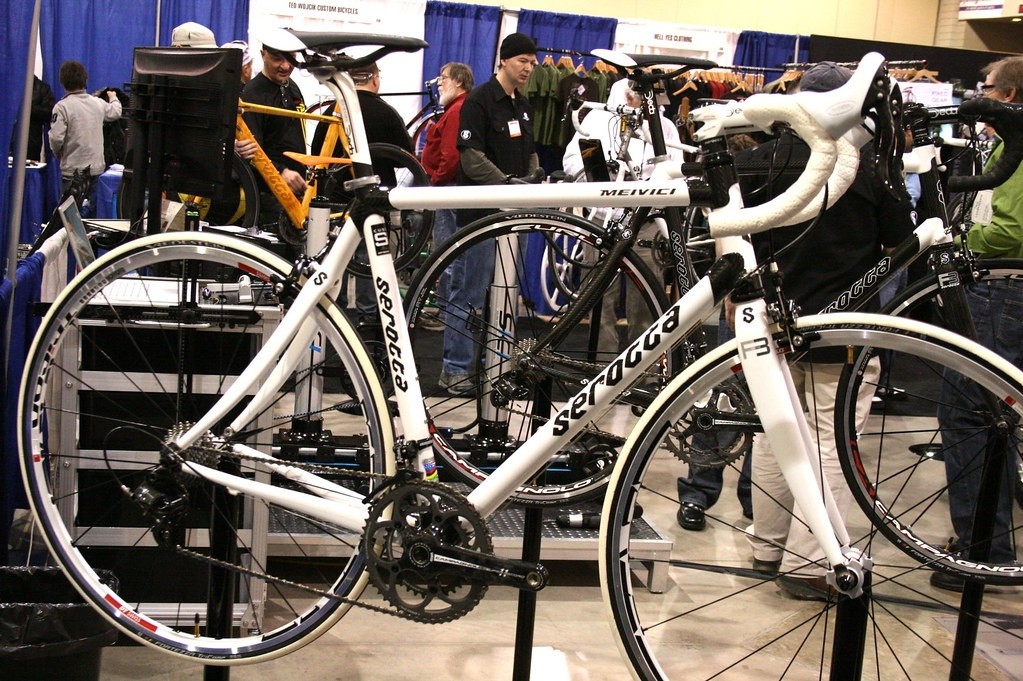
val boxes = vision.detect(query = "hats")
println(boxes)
[256,26,308,52]
[219,39,252,66]
[172,21,219,48]
[500,33,536,61]
[800,61,853,93]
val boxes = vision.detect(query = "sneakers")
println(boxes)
[419,310,446,332]
[439,370,475,396]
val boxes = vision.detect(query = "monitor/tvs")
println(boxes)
[124,47,244,182]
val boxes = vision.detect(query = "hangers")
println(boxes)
[535,48,942,96]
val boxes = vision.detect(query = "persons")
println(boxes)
[48,61,122,232]
[168,19,1023,602]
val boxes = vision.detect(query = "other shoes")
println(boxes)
[773,570,840,602]
[753,556,784,574]
[929,543,1023,592]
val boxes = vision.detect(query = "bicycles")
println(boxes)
[16,29,1023,681]
[541,93,668,317]
[114,59,435,279]
[399,45,1023,587]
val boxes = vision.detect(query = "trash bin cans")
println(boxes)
[0,565,118,681]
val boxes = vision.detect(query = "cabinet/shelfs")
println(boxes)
[45,276,284,632]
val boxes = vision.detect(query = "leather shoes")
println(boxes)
[873,386,906,399]
[744,511,753,518]
[677,501,705,530]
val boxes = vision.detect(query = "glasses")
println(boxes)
[437,75,450,84]
[981,83,1000,94]
